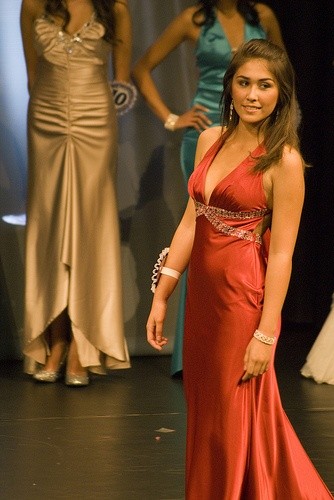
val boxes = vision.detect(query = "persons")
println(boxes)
[146,39,334,500]
[21,0,137,385]
[134,0,288,375]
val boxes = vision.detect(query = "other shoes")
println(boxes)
[33,344,68,382]
[65,371,89,385]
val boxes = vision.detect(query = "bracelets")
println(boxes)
[161,267,182,280]
[164,113,178,132]
[253,329,276,345]
